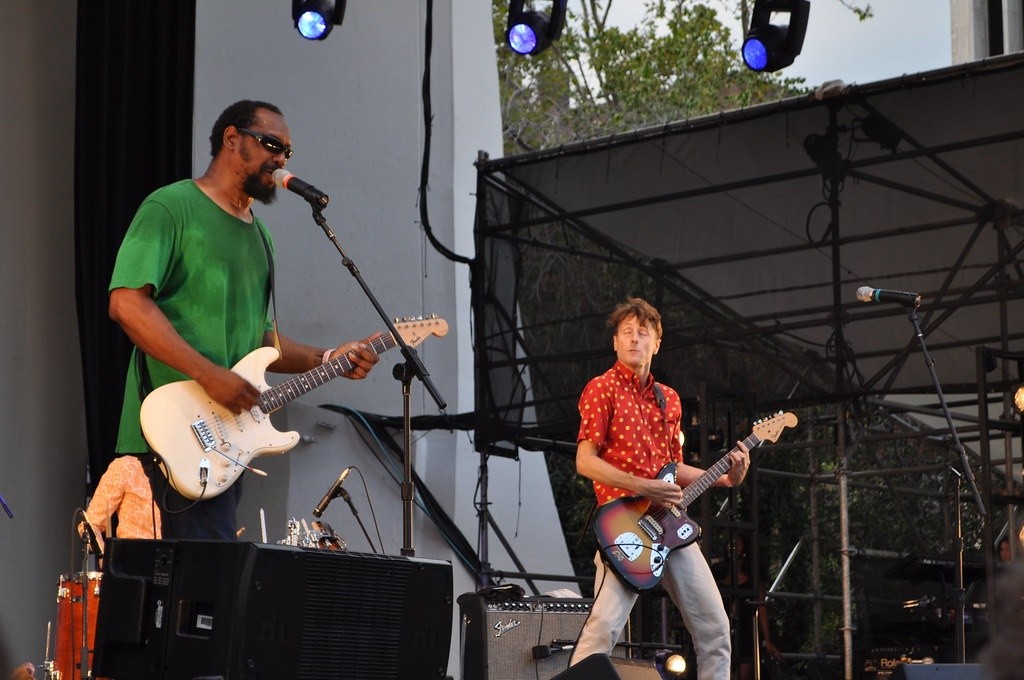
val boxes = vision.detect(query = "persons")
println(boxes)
[569,298,751,680]
[76,456,162,570]
[711,531,782,680]
[998,538,1011,561]
[106,99,381,540]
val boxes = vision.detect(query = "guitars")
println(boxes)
[139,310,449,504]
[590,410,799,595]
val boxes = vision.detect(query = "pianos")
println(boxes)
[887,551,1011,663]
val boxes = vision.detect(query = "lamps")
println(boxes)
[741,0,810,73]
[654,649,691,680]
[504,0,568,56]
[291,0,347,40]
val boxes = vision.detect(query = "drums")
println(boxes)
[51,571,104,680]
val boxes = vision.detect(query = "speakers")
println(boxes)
[91,538,455,679]
[456,591,664,680]
[888,663,992,680]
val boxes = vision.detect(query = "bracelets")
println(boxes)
[723,475,733,488]
[322,349,337,364]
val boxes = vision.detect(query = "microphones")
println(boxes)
[272,168,330,206]
[80,510,104,559]
[856,286,921,305]
[531,645,576,659]
[210,446,268,477]
[313,467,351,518]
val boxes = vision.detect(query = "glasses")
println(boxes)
[238,126,294,160]
